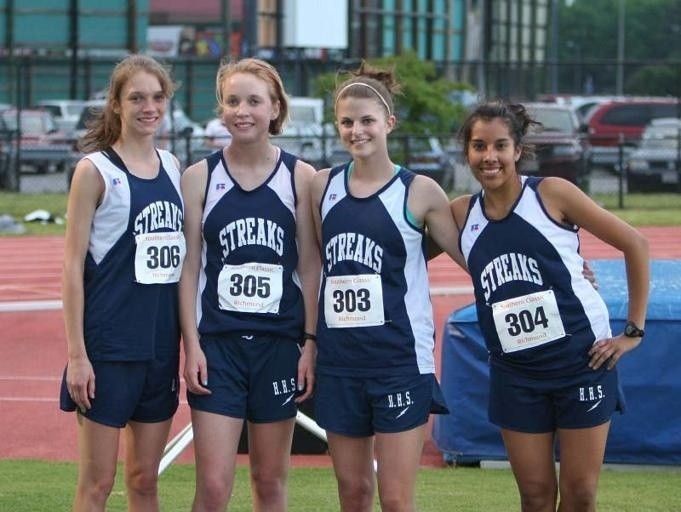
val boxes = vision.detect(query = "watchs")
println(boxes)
[623,318,644,339]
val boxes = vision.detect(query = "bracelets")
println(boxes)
[302,332,316,346]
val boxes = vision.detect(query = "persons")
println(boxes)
[179,50,323,510]
[55,52,187,512]
[423,93,650,511]
[308,55,600,512]
[65,105,102,189]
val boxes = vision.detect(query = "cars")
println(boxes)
[333,121,455,191]
[1,88,202,187]
[537,91,681,167]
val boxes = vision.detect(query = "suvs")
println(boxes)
[517,101,586,182]
[626,117,681,192]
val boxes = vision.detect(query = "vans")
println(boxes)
[205,95,333,166]
